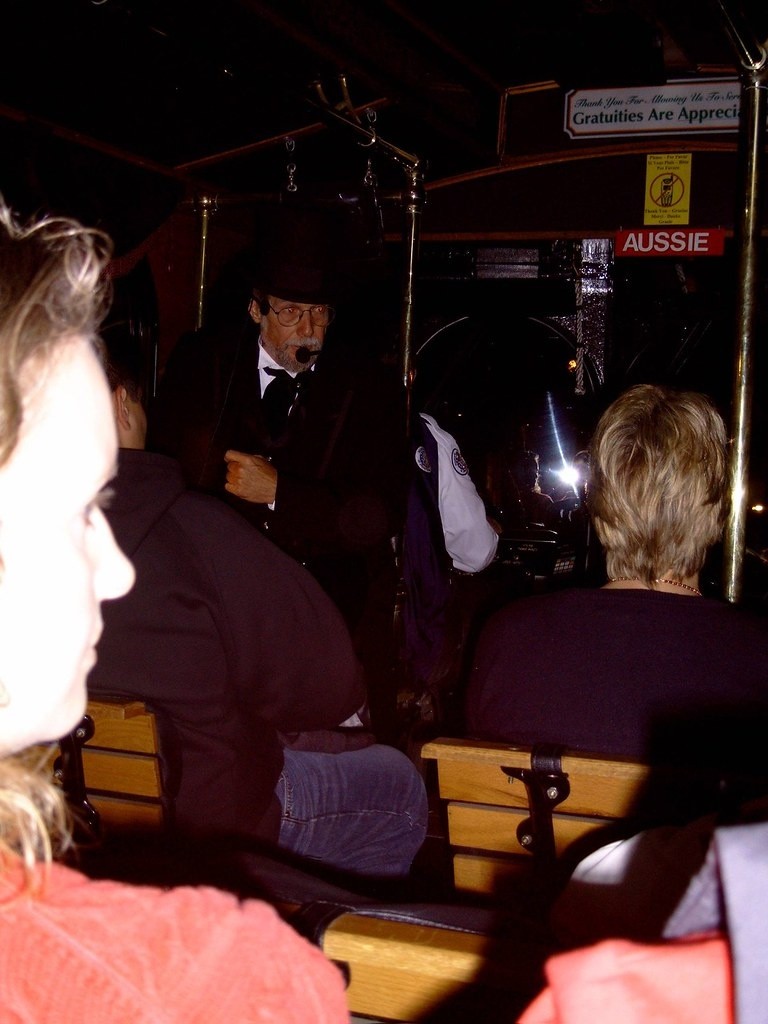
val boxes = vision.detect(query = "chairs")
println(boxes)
[5,693,184,889]
[419,735,768,925]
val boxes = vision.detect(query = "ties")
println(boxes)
[261,369,297,440]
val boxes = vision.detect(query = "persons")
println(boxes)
[512,817,768,1024]
[2,199,353,1023]
[464,382,766,762]
[85,354,431,900]
[396,359,503,736]
[147,245,388,606]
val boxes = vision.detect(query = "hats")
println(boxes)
[249,204,346,304]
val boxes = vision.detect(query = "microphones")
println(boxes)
[295,347,322,364]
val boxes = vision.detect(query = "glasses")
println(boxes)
[251,297,338,326]
